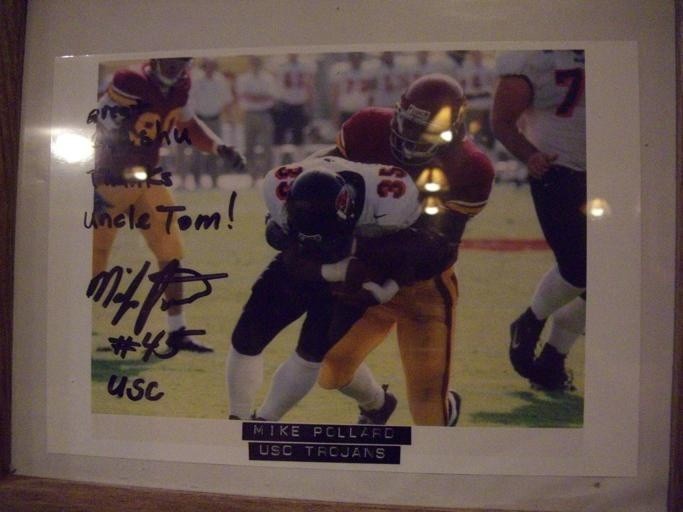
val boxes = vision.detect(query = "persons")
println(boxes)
[232,52,320,189]
[174,58,240,190]
[485,49,586,398]
[328,51,495,151]
[90,58,250,356]
[223,155,422,427]
[302,69,498,425]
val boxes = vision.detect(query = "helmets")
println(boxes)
[389,73,467,170]
[151,57,194,87]
[288,169,355,244]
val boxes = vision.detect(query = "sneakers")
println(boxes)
[358,392,397,424]
[508,307,576,390]
[166,327,214,352]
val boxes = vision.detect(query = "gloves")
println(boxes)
[217,144,247,173]
[321,256,401,310]
